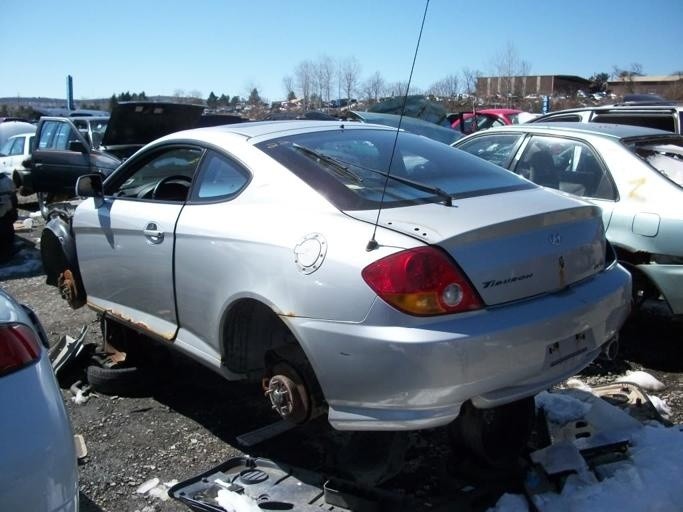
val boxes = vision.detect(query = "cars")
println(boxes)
[0,131,37,188]
[449,108,533,137]
[41,121,636,432]
[446,119,683,326]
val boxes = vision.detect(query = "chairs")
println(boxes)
[530,149,559,190]
